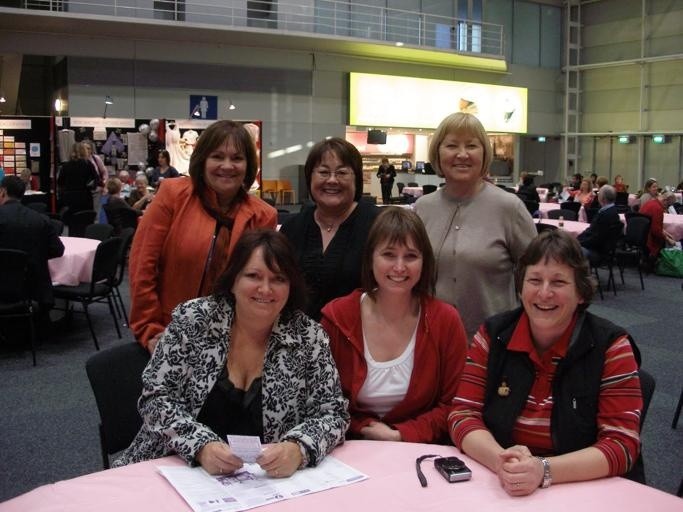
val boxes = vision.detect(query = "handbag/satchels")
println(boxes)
[656,247,683,277]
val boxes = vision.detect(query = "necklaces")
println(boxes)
[316,210,347,232]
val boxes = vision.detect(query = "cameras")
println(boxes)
[433,456,472,483]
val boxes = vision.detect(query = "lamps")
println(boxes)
[226,97,240,113]
[188,104,204,119]
[101,94,116,120]
[53,87,73,117]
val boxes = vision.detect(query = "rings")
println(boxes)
[274,469,279,476]
[516,483,521,493]
[220,468,222,475]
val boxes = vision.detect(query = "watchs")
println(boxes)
[540,457,552,489]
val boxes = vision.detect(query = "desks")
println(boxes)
[0,433,683,512]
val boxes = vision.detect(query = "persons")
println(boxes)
[660,185,683,215]
[118,170,134,185]
[81,140,108,223]
[576,184,620,239]
[574,178,596,207]
[319,208,471,441]
[0,174,65,347]
[376,158,397,203]
[128,118,278,360]
[447,229,645,499]
[273,135,391,324]
[102,178,144,228]
[149,149,179,187]
[517,174,541,203]
[199,96,209,119]
[612,175,629,192]
[638,191,676,257]
[515,171,528,193]
[547,184,563,203]
[112,226,351,479]
[413,112,537,344]
[130,174,155,210]
[56,142,99,227]
[564,173,582,203]
[591,173,600,188]
[639,178,658,208]
[0,165,5,186]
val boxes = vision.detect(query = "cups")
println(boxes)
[664,184,670,192]
[671,184,676,193]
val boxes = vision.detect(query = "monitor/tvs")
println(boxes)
[402,161,411,168]
[416,162,425,169]
[367,128,386,144]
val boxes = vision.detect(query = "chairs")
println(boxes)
[49,235,123,350]
[0,247,47,368]
[408,179,419,187]
[612,187,631,209]
[560,202,582,214]
[586,223,622,302]
[107,221,137,333]
[69,206,96,231]
[584,193,602,216]
[82,223,117,240]
[629,357,659,485]
[623,213,653,291]
[396,181,406,198]
[85,337,169,472]
[546,209,577,223]
[421,185,439,196]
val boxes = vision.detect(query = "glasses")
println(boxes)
[314,166,353,181]
[158,149,166,153]
[596,192,601,195]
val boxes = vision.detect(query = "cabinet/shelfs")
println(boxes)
[0,112,58,218]
